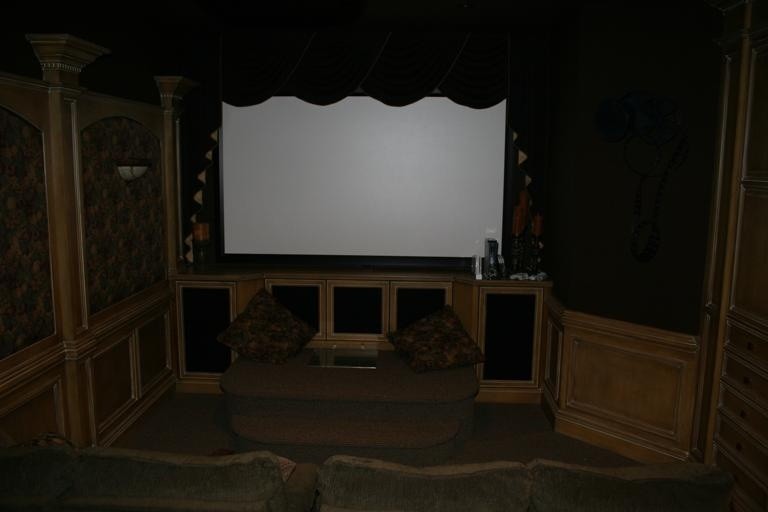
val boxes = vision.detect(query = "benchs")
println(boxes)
[220,356,479,448]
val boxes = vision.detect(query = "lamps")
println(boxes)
[116,161,151,182]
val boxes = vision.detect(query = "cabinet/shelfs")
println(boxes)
[453,281,551,404]
[261,274,452,368]
[693,0,768,512]
[174,272,264,396]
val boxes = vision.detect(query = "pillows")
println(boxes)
[2,450,736,509]
[389,305,486,374]
[217,287,317,367]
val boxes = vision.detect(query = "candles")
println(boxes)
[513,191,544,236]
